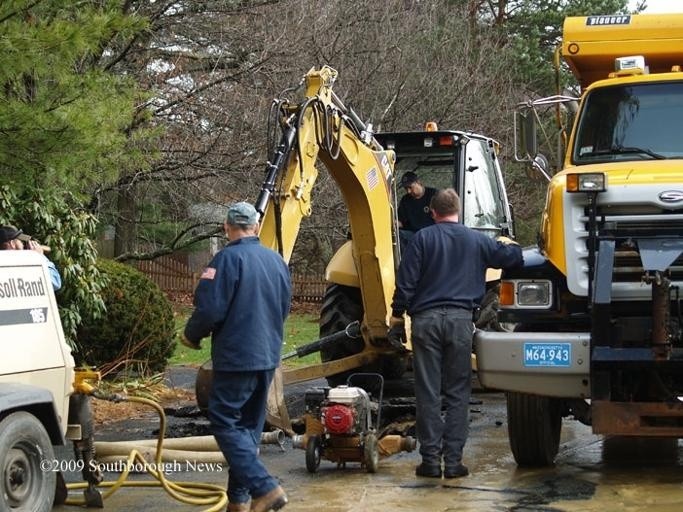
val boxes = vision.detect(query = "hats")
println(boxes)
[0,225,31,242]
[226,202,259,226]
[400,171,417,189]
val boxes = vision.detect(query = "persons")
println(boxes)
[396,170,438,255]
[177,202,294,512]
[386,189,544,477]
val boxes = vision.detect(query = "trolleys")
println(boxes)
[302,368,388,475]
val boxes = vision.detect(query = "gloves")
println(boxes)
[386,323,407,345]
[498,236,519,246]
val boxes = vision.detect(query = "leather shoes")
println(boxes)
[253,487,288,511]
[444,465,470,478]
[416,463,442,476]
[225,499,251,512]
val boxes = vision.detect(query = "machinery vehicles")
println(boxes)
[460,10,683,472]
[190,60,527,438]
